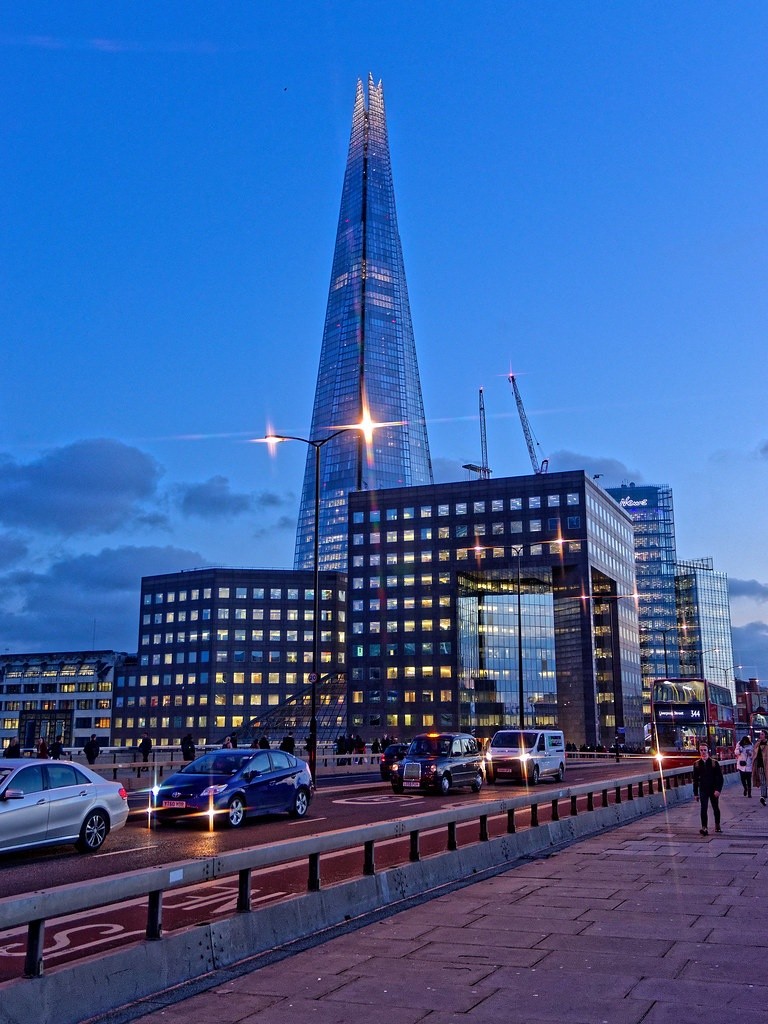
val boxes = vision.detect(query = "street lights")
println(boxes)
[639,624,688,678]
[263,420,396,790]
[473,538,567,731]
[679,648,719,679]
[708,664,743,688]
[580,593,639,762]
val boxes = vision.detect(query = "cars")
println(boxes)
[379,743,411,782]
[150,748,316,829]
[0,755,130,854]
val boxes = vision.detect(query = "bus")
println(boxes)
[648,677,738,772]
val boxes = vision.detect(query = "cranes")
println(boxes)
[477,387,492,478]
[505,375,551,474]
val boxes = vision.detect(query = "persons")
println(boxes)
[279,730,295,755]
[32,736,68,759]
[336,733,366,764]
[138,731,152,772]
[222,732,238,749]
[371,734,398,764]
[250,734,270,750]
[565,740,644,758]
[752,729,768,806]
[180,733,195,761]
[692,742,723,835]
[83,734,100,765]
[734,736,755,798]
[4,737,21,758]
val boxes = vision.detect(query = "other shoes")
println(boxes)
[715,824,721,832]
[700,826,708,836]
[744,789,747,796]
[748,792,751,797]
[760,797,767,806]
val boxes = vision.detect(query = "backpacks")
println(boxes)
[222,742,229,749]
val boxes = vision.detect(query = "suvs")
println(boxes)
[391,732,484,796]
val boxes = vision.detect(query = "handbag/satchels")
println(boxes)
[740,761,746,766]
[181,741,192,752]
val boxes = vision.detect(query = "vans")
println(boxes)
[485,729,567,785]
